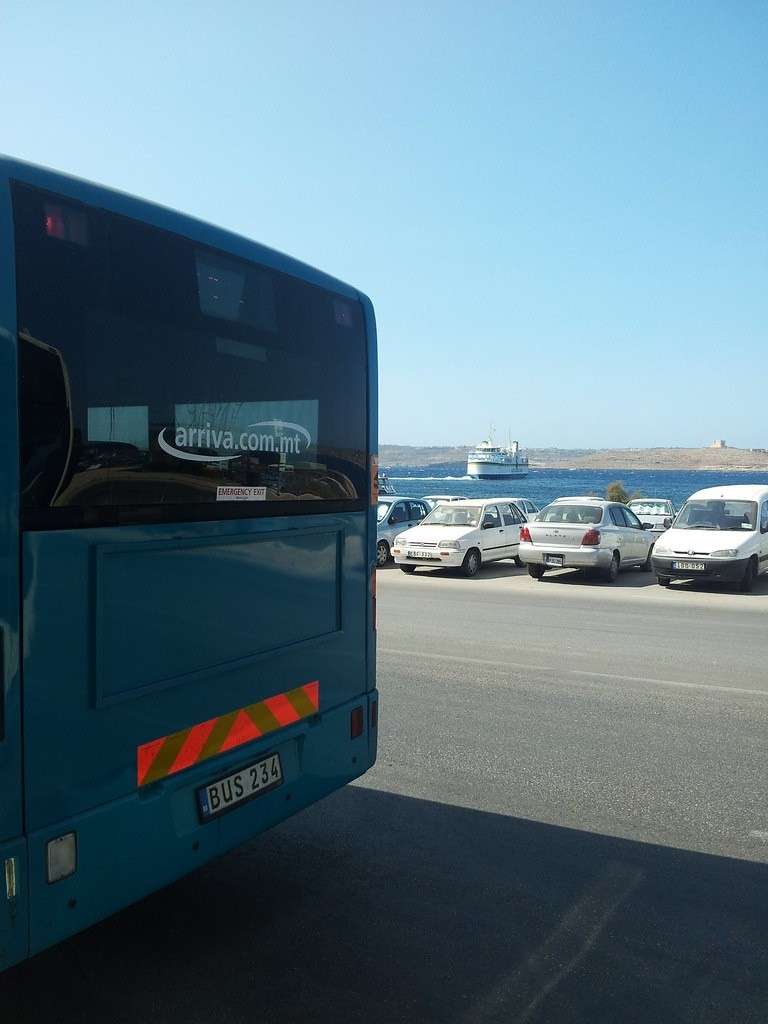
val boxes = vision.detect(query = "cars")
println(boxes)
[378,493,433,567]
[626,497,676,545]
[390,499,530,576]
[651,484,768,593]
[517,500,655,583]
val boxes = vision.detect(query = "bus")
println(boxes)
[4,149,384,959]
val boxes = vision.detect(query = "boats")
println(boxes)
[466,429,529,480]
